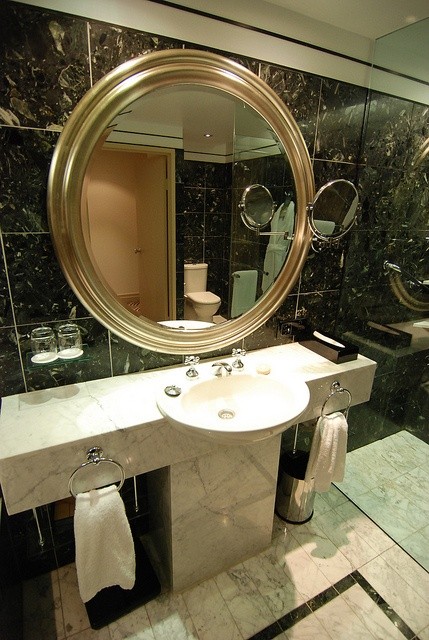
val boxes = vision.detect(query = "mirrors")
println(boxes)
[237,182,288,240]
[48,47,318,360]
[311,177,362,245]
[392,144,428,318]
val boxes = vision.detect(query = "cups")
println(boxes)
[59,323,83,358]
[30,327,57,363]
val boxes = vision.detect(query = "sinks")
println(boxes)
[180,375,296,432]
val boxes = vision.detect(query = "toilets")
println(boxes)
[183,263,221,323]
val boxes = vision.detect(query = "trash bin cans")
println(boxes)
[275,449,316,525]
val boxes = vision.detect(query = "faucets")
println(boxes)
[212,361,232,376]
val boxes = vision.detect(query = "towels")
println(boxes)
[228,267,259,318]
[71,481,141,605]
[302,409,350,495]
